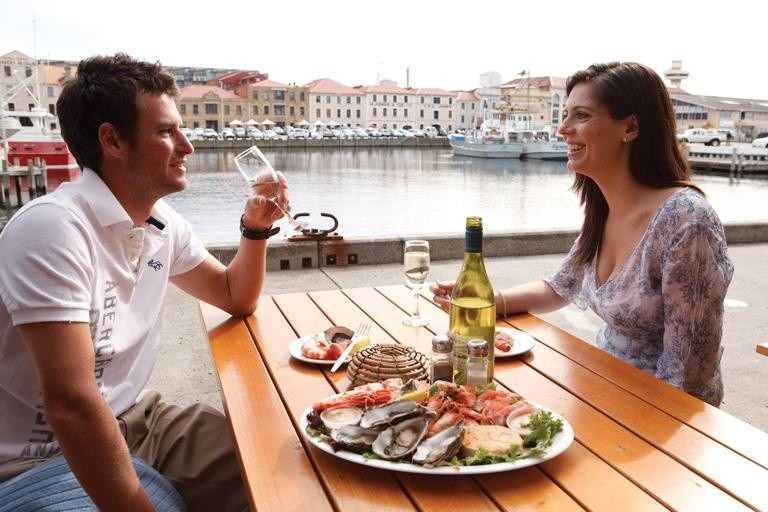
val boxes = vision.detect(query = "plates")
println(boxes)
[301,378,575,477]
[495,325,538,358]
[287,330,377,365]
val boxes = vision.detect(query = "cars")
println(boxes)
[179,124,439,142]
[749,137,767,151]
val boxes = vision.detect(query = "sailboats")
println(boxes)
[445,67,569,160]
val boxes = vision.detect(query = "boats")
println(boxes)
[0,106,78,181]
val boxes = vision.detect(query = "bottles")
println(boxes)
[447,215,496,387]
[465,339,490,385]
[428,334,454,385]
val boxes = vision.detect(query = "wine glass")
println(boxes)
[399,241,434,328]
[232,145,309,237]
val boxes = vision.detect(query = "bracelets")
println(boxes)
[239,213,280,241]
[497,290,508,318]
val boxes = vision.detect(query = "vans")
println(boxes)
[712,128,736,142]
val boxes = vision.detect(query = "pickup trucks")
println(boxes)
[676,127,724,148]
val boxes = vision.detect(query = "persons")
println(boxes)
[0,53,292,512]
[428,59,733,408]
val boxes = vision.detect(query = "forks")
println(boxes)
[331,323,372,373]
